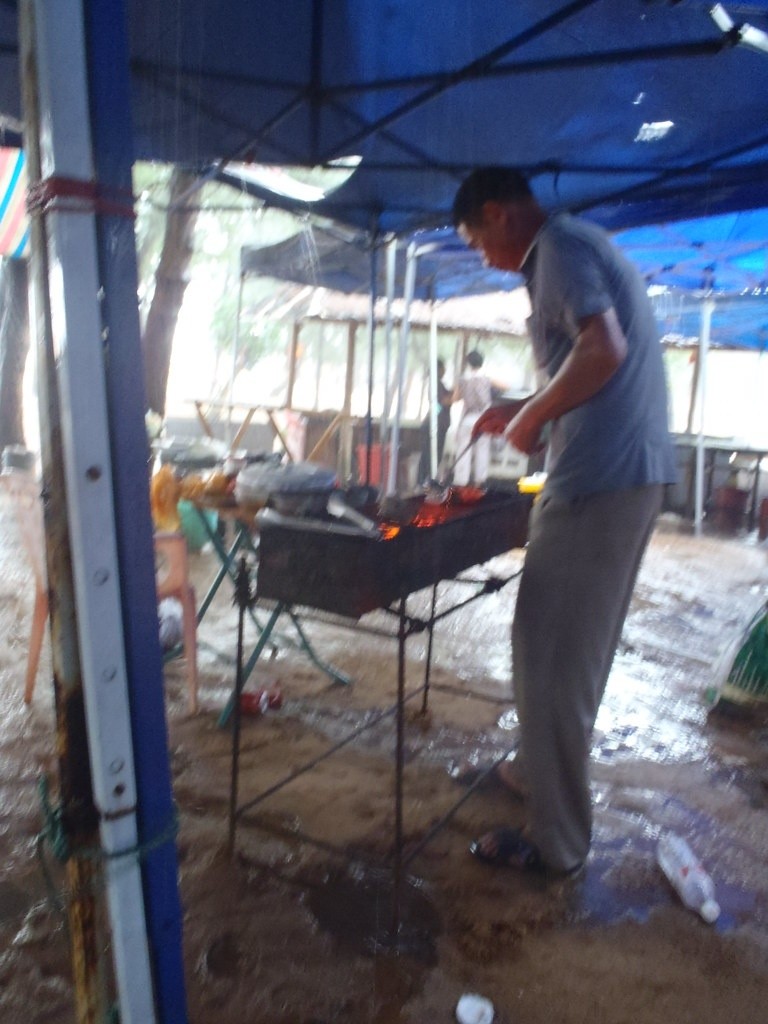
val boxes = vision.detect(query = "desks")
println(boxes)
[671,443,768,533]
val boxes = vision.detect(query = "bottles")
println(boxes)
[655,836,722,925]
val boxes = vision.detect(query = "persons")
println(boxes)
[415,352,510,488]
[451,163,680,877]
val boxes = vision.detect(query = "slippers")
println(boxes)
[450,761,525,803]
[469,823,583,881]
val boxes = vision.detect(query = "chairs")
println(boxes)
[0,473,199,717]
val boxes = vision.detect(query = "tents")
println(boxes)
[0,1,767,1024]
